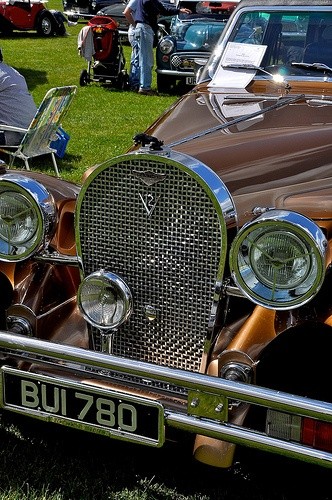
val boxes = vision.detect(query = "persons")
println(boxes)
[135,0,193,96]
[123,0,138,90]
[0,50,38,150]
[38,0,78,36]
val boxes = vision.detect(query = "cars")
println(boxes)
[154,17,264,93]
[0,0,53,37]
[63,0,307,45]
[2,0,332,500]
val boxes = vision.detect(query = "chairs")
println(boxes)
[0,85,78,178]
[304,39,332,69]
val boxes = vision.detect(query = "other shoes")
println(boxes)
[138,88,159,96]
[67,20,78,27]
[63,32,71,36]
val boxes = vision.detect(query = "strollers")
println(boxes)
[78,15,130,90]
